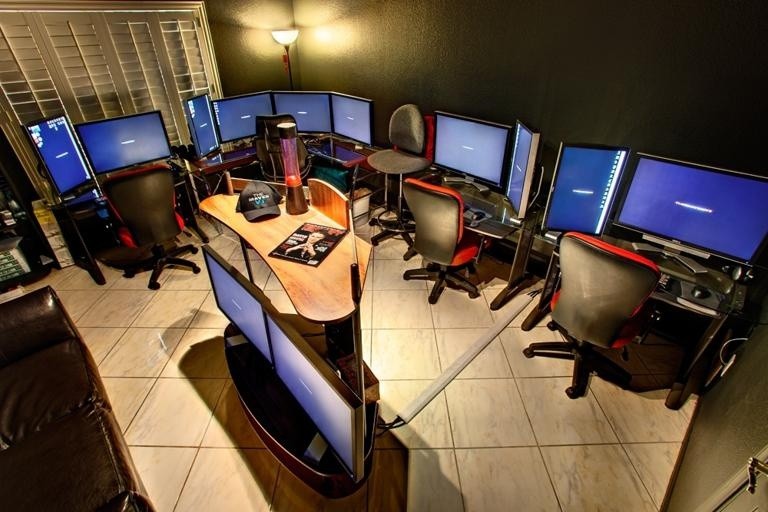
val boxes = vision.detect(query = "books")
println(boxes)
[268,222,350,268]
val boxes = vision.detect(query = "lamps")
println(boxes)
[271,30,299,90]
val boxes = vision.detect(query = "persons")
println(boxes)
[283,229,327,260]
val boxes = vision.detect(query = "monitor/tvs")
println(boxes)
[430,110,539,219]
[259,303,366,484]
[22,109,175,199]
[185,91,372,159]
[541,109,768,274]
[199,243,274,369]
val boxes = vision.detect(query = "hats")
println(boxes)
[236,180,283,222]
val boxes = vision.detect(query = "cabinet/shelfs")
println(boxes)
[659,319,768,512]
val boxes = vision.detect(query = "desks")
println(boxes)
[0,218,40,287]
[199,172,385,497]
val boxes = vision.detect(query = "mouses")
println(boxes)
[693,285,708,299]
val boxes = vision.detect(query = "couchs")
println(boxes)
[0,285,156,512]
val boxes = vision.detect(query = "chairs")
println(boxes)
[104,163,204,294]
[367,104,432,255]
[254,114,311,185]
[522,229,667,403]
[399,176,484,303]
